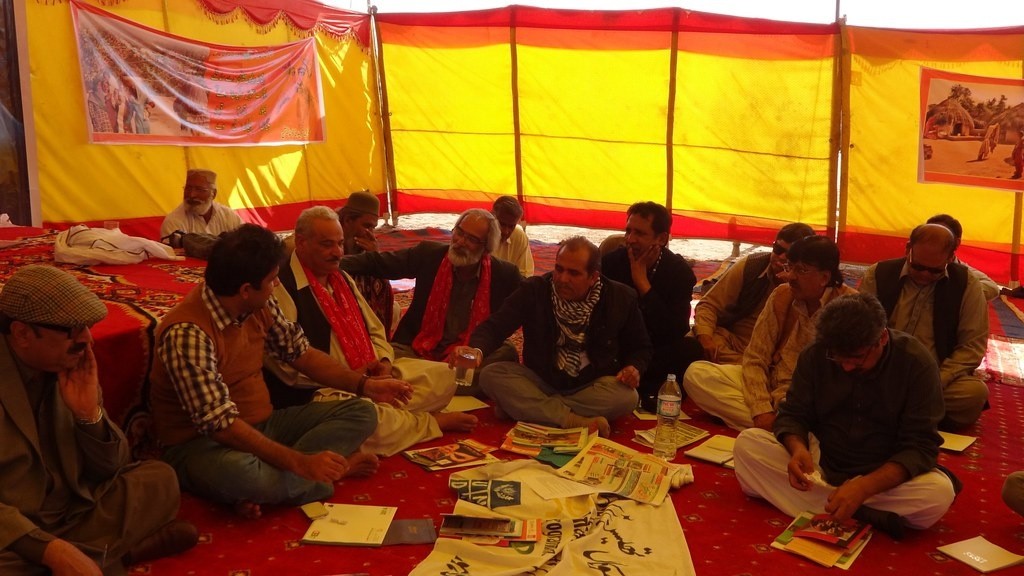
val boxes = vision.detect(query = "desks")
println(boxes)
[0,224,215,451]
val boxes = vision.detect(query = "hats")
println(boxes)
[0,265,108,327]
[344,188,378,216]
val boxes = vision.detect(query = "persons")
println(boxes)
[0,262,198,576]
[490,196,535,278]
[338,208,527,399]
[599,201,704,408]
[146,193,479,519]
[684,214,1000,433]
[159,168,245,247]
[419,443,476,462]
[733,291,964,539]
[455,237,647,438]
[88,68,155,133]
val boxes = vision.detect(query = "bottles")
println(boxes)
[653,374,681,459]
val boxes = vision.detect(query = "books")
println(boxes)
[301,503,398,545]
[771,511,873,570]
[937,535,1024,573]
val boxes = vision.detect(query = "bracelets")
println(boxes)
[355,372,370,398]
[74,405,104,425]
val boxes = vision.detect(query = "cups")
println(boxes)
[455,348,476,386]
[104,221,119,229]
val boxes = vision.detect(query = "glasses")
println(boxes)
[910,248,947,273]
[183,186,212,194]
[824,339,877,367]
[452,226,487,246]
[783,264,822,275]
[33,324,84,339]
[772,241,788,256]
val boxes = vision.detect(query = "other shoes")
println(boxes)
[126,521,199,565]
[561,412,611,438]
[855,505,903,540]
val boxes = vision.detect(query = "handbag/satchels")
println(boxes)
[183,232,221,260]
